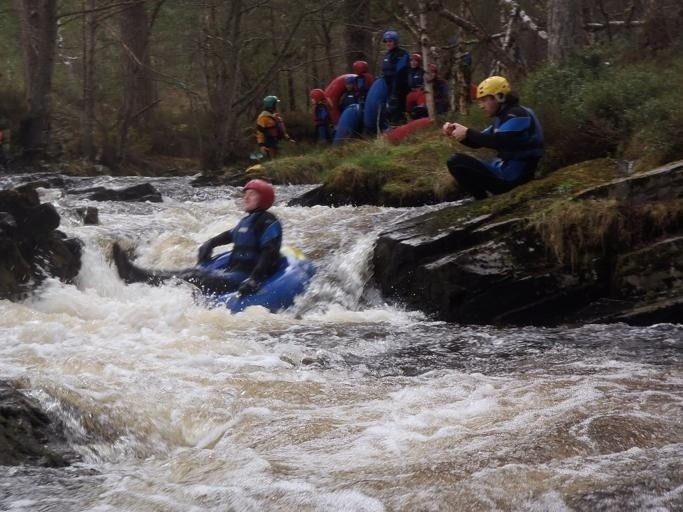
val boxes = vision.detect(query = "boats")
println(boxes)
[187,244,315,319]
[381,78,478,144]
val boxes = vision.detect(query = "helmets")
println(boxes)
[353,61,368,76]
[430,64,437,72]
[344,77,355,84]
[263,96,280,108]
[244,179,275,211]
[477,76,510,103]
[412,54,421,63]
[309,88,325,102]
[383,31,399,42]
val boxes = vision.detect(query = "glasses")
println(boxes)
[383,39,392,43]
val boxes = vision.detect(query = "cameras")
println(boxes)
[444,123,459,137]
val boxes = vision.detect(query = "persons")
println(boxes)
[442,76,546,200]
[113,179,283,296]
[257,31,472,163]
